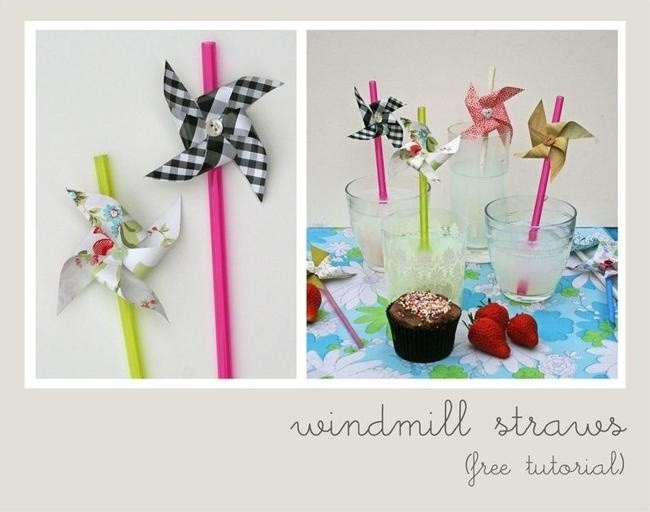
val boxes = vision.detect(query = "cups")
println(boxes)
[346,173,434,271]
[449,122,511,259]
[489,193,578,304]
[379,206,467,309]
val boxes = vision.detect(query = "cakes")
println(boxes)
[386,290,462,361]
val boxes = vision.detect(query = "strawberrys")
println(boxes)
[466,299,541,359]
[307,282,322,322]
